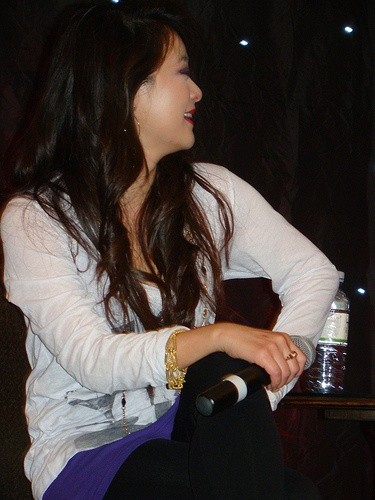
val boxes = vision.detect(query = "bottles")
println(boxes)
[304,272,349,394]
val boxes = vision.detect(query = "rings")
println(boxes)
[285,350,298,361]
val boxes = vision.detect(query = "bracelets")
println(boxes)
[166,328,188,391]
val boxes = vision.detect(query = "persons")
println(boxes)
[0,1,341,500]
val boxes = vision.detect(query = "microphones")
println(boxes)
[194,334,315,418]
